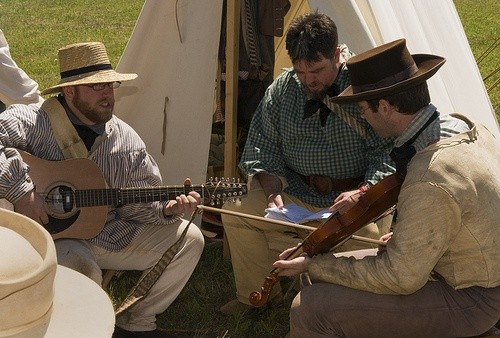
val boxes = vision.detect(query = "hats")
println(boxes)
[0,207,115,338]
[38,41,138,95]
[327,37,447,104]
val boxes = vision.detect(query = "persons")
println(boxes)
[0,28,205,338]
[273,37,500,338]
[221,13,400,315]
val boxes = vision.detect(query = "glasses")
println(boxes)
[82,80,122,91]
[357,106,370,120]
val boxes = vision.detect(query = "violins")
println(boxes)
[247,169,405,308]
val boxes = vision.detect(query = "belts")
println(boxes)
[287,167,365,192]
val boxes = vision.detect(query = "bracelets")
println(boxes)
[268,193,275,200]
[358,187,365,193]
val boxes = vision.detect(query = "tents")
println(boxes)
[112,0,500,242]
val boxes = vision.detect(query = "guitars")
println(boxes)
[0,147,247,241]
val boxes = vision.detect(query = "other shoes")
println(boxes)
[220,288,283,312]
[115,326,185,338]
